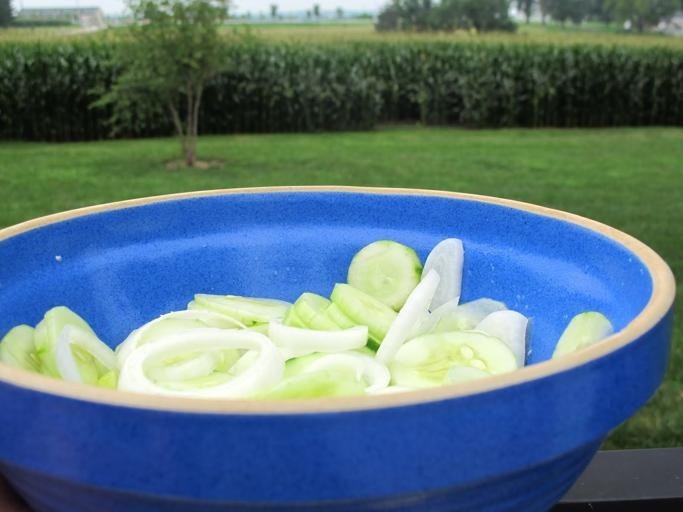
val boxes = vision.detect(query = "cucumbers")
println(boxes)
[0,239,613,400]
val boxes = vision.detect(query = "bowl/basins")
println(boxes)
[0,185,677,511]
